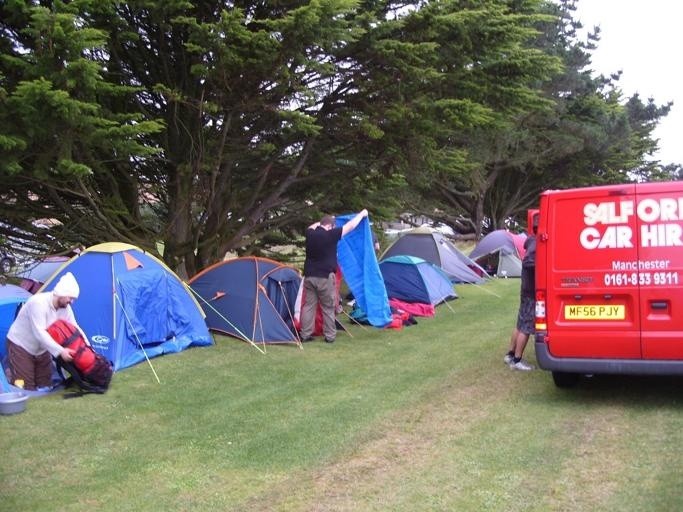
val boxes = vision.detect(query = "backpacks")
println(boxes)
[43,319,116,402]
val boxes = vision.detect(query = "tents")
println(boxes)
[377,254,458,309]
[183,255,366,348]
[377,226,492,285]
[465,228,528,278]
[14,255,72,296]
[33,241,219,377]
[0,271,90,391]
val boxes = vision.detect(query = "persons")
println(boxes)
[371,229,379,256]
[0,283,67,398]
[502,222,537,371]
[297,208,369,344]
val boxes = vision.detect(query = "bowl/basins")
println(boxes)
[0,392,29,415]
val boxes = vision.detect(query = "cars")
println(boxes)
[0,250,16,273]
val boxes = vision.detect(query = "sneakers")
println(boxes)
[503,353,538,372]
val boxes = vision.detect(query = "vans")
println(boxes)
[527,181,683,388]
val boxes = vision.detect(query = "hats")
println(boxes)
[53,272,80,298]
